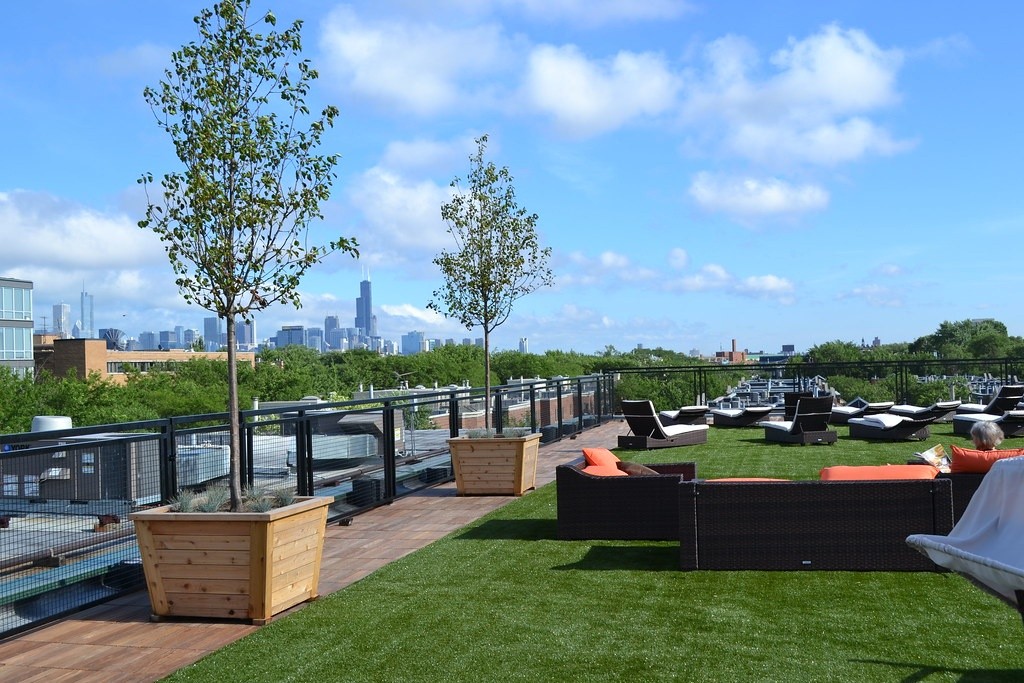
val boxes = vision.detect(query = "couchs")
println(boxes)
[557,456,696,541]
[681,479,956,575]
[907,459,988,529]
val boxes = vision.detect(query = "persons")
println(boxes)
[949,421,1005,467]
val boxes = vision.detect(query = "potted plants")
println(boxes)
[126,2,361,626]
[425,133,555,496]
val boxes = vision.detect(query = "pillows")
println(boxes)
[582,448,620,466]
[616,462,658,477]
[820,465,940,480]
[581,465,626,477]
[949,444,1023,472]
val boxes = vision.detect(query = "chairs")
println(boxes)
[710,406,771,427]
[920,374,1011,405]
[952,408,1024,440]
[618,399,708,447]
[660,406,708,427]
[722,378,821,407]
[891,402,960,422]
[957,384,1023,416]
[833,401,893,424]
[847,414,935,443]
[761,396,833,446]
[784,392,811,419]
